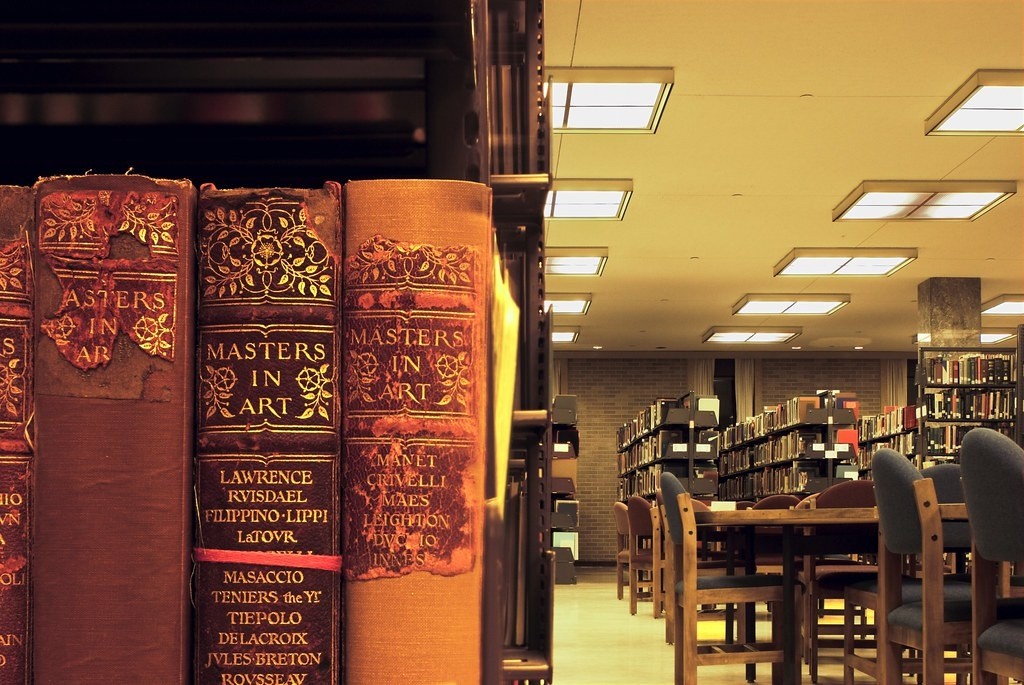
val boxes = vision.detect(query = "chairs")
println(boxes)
[611,426,1024,685]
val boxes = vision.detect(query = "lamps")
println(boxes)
[538,65,1023,345]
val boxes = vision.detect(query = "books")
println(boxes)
[0,173,531,685]
[720,389,859,502]
[553,394,579,562]
[859,354,1016,481]
[614,395,719,579]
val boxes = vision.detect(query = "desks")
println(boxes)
[697,503,966,685]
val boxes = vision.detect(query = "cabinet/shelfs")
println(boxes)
[552,426,579,585]
[616,347,1024,593]
[491,172,553,685]
